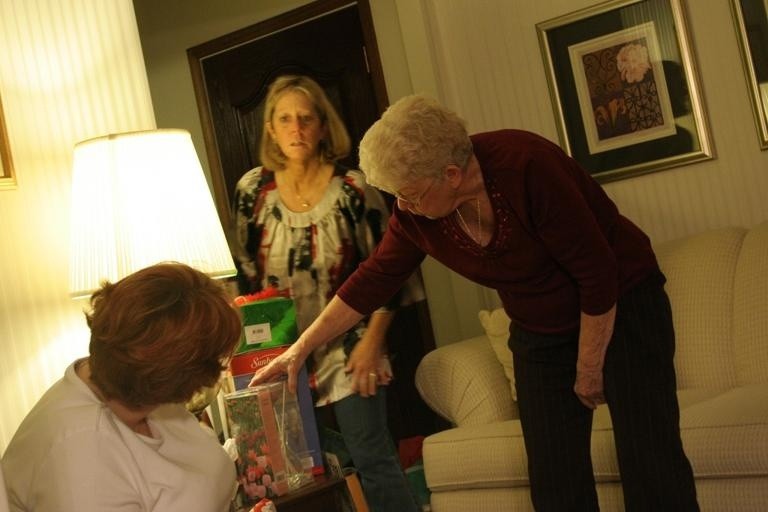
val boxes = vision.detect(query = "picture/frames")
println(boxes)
[535,0,718,185]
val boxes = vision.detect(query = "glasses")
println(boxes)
[397,175,438,205]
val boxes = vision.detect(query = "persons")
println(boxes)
[0,260,250,511]
[251,91,701,511]
[227,73,428,512]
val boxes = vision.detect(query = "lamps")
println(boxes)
[67,129,238,301]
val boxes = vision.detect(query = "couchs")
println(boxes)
[414,217,768,511]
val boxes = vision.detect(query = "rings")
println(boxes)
[368,373,378,378]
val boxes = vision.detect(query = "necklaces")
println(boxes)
[456,172,485,245]
[287,175,317,208]
